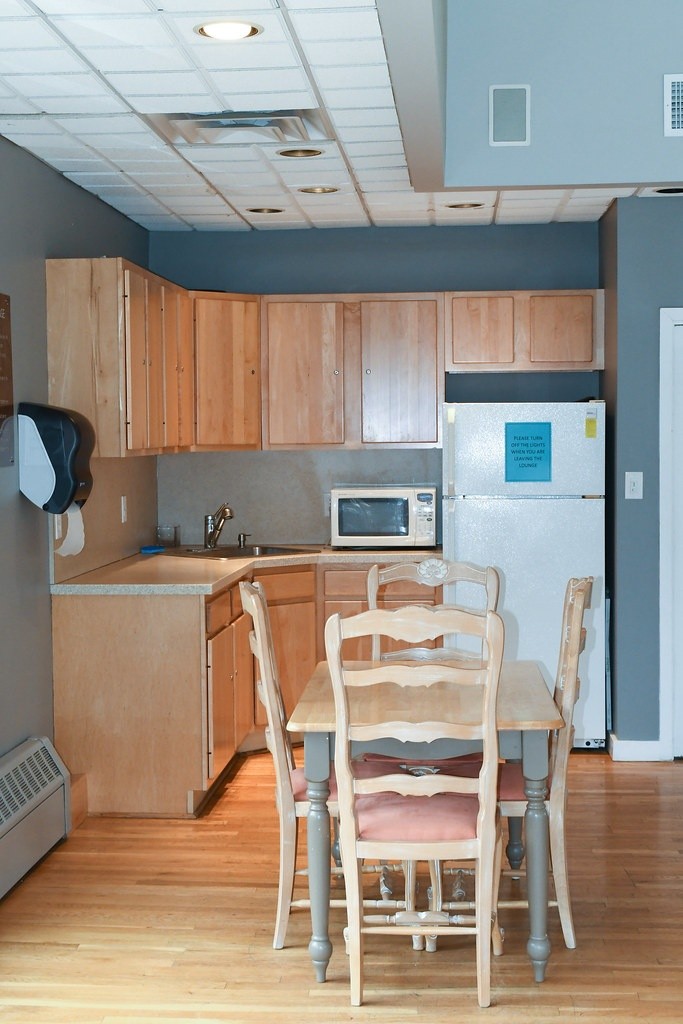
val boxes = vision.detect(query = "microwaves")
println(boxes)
[329,487,436,546]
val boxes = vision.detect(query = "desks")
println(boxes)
[286,660,566,982]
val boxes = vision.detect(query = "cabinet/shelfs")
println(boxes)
[190,289,262,451]
[164,274,194,455]
[261,292,445,452]
[253,564,317,726]
[46,256,163,457]
[444,288,604,374]
[49,579,253,819]
[318,564,442,663]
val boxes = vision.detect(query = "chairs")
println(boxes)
[238,580,425,951]
[324,603,505,1008]
[426,576,594,952]
[362,558,499,762]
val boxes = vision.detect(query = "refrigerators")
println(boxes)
[441,403,605,750]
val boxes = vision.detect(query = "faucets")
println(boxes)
[203,502,233,548]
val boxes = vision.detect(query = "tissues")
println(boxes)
[16,401,95,554]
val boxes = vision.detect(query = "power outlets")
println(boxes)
[624,471,644,500]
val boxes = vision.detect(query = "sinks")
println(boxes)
[185,544,304,558]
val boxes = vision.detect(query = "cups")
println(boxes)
[157,525,180,547]
